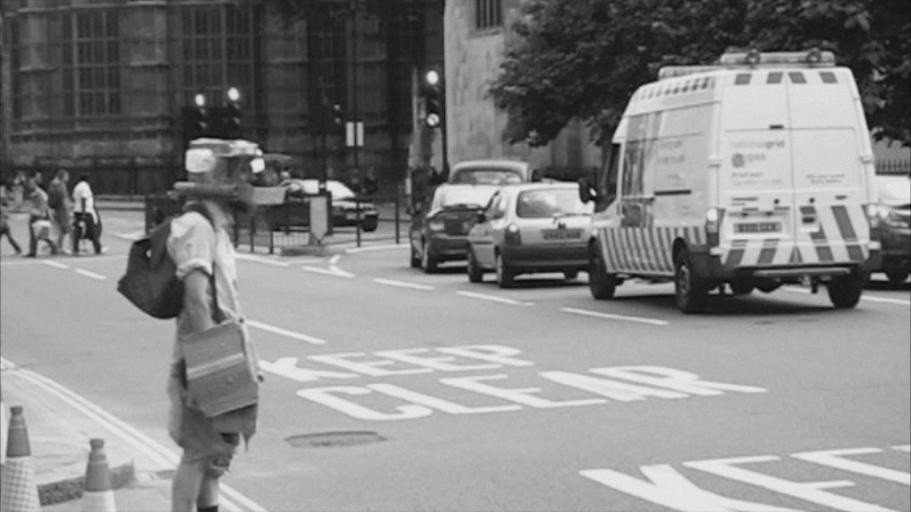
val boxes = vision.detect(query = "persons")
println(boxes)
[163,141,261,512]
[1,184,23,257]
[26,177,59,257]
[69,173,109,256]
[48,167,75,255]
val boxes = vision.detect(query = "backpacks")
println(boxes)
[115,200,219,322]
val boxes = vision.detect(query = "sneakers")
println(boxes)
[10,248,72,258]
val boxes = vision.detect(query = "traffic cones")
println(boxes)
[80,438,116,512]
[0,404,42,512]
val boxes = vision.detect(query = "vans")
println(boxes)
[579,49,881,317]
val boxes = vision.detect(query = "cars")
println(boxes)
[864,174,911,286]
[407,159,597,289]
[274,179,380,232]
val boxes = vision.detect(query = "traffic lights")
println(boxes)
[330,97,344,130]
[225,87,242,129]
[425,70,443,127]
[193,91,207,132]
